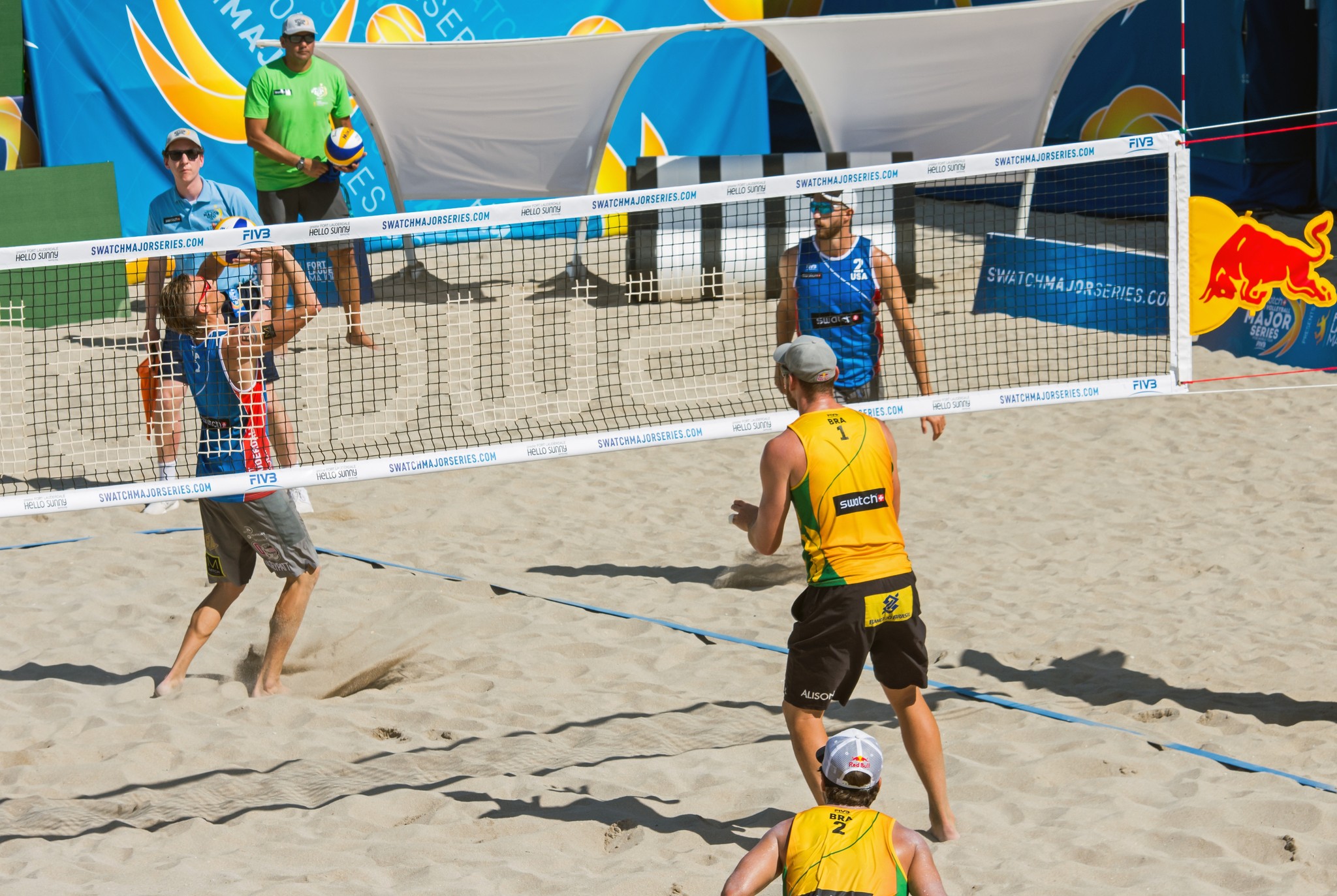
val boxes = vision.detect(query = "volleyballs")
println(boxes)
[324,127,364,167]
[211,216,258,268]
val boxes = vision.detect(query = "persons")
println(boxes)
[774,184,945,441]
[143,15,385,702]
[725,335,959,841]
[718,729,948,896]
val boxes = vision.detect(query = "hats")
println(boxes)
[282,13,318,35]
[165,128,201,149]
[801,185,857,212]
[773,334,837,382]
[815,727,883,789]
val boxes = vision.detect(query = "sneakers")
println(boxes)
[287,487,314,514]
[142,499,180,516]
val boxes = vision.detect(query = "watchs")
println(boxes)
[259,299,273,308]
[296,157,305,171]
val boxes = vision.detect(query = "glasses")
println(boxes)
[288,34,315,43]
[780,365,790,378]
[197,275,212,308]
[167,149,199,160]
[810,201,844,215]
